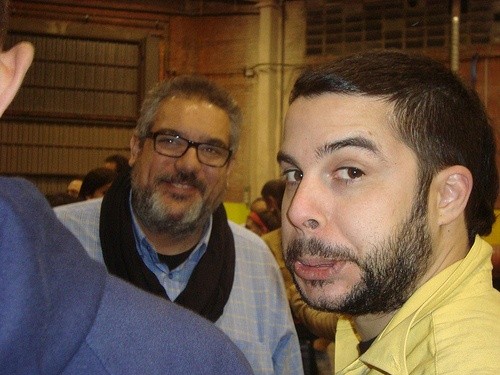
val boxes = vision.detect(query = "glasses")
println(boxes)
[146,132,232,167]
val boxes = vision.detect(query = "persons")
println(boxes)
[46,154,129,208]
[1,1,253,374]
[53,75,304,374]
[218,179,339,374]
[277,48,500,375]
